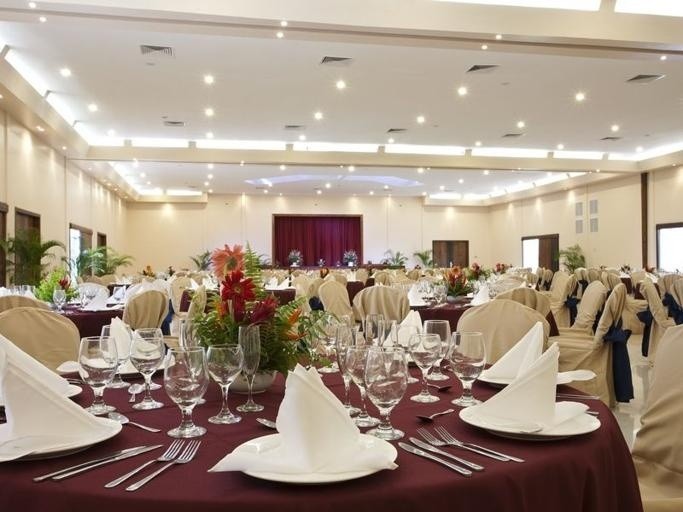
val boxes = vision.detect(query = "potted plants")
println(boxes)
[31,265,83,310]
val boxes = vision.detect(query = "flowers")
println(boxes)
[465,262,491,283]
[441,266,479,298]
[178,239,341,384]
[287,249,303,265]
[492,262,513,275]
[342,248,359,264]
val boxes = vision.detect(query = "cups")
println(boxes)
[181,324,201,351]
[423,281,431,291]
[129,328,166,410]
[449,332,486,408]
[22,285,29,296]
[235,324,264,412]
[78,336,119,417]
[179,319,201,351]
[335,327,362,415]
[53,290,66,314]
[408,332,443,404]
[344,344,383,428]
[363,314,384,347]
[164,346,210,440]
[14,286,22,296]
[28,286,36,296]
[426,287,431,302]
[396,324,423,386]
[332,316,351,369]
[364,348,408,441]
[421,320,452,383]
[306,328,319,374]
[85,284,99,302]
[99,325,132,389]
[113,287,125,309]
[322,314,336,364]
[417,286,423,300]
[204,343,245,425]
[377,319,397,348]
[434,285,445,308]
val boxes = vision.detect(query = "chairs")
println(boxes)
[547,281,629,413]
[307,277,325,311]
[456,299,551,366]
[538,269,571,304]
[0,294,54,315]
[557,280,609,341]
[630,323,682,512]
[317,279,356,326]
[638,276,676,387]
[630,271,645,296]
[353,284,410,323]
[657,273,683,300]
[121,290,171,332]
[324,272,347,290]
[549,275,579,328]
[187,285,208,320]
[586,267,601,284]
[0,305,82,377]
[606,271,650,337]
[662,279,683,325]
[168,276,192,337]
[71,282,109,297]
[490,285,552,332]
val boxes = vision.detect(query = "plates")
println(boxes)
[65,384,83,398]
[13,417,123,462]
[458,404,602,442]
[108,356,177,378]
[476,373,573,389]
[231,431,398,486]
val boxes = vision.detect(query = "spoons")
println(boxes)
[107,412,161,433]
[426,381,452,392]
[255,416,277,432]
[415,407,455,425]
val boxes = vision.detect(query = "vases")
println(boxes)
[348,262,353,267]
[229,370,279,395]
[293,262,298,268]
[445,297,464,303]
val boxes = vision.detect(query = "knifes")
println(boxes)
[398,442,473,476]
[52,443,164,483]
[33,445,146,483]
[409,437,485,472]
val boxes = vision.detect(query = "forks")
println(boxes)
[125,439,202,493]
[417,426,510,462]
[105,438,186,491]
[433,424,526,464]
